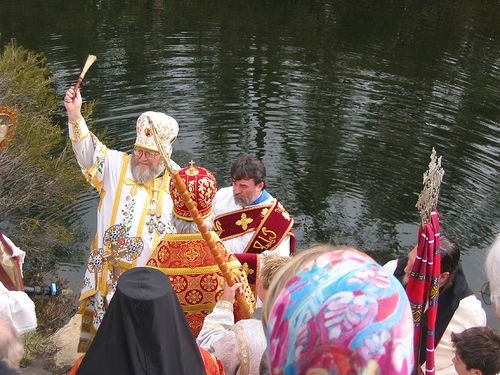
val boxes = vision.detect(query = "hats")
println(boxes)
[168,159,218,221]
[133,111,180,154]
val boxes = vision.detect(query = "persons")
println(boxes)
[0,233,500,375]
[146,161,255,340]
[64,86,183,353]
[212,157,295,308]
